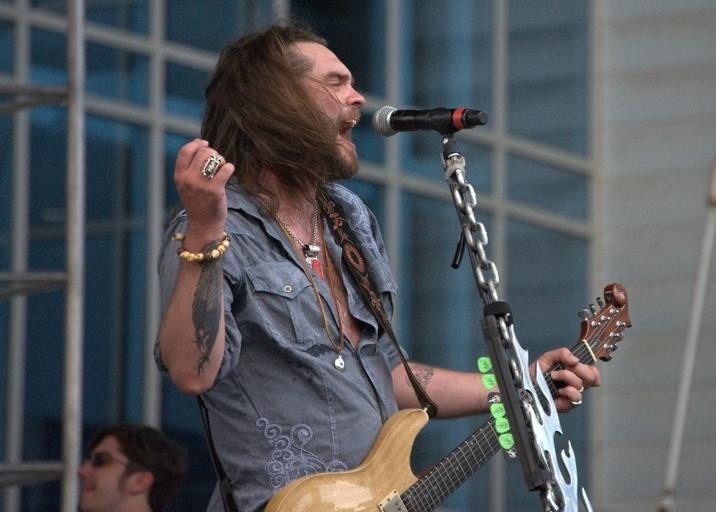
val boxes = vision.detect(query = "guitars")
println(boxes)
[263,283,632,512]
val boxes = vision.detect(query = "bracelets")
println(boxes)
[173,230,231,263]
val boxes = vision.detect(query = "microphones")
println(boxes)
[370,104,489,137]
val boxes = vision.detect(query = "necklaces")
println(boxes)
[277,200,326,280]
[273,213,345,370]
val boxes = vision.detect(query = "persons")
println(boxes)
[154,11,602,512]
[78,421,183,511]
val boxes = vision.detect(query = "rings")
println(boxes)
[202,154,222,178]
[571,400,583,405]
[578,385,584,392]
